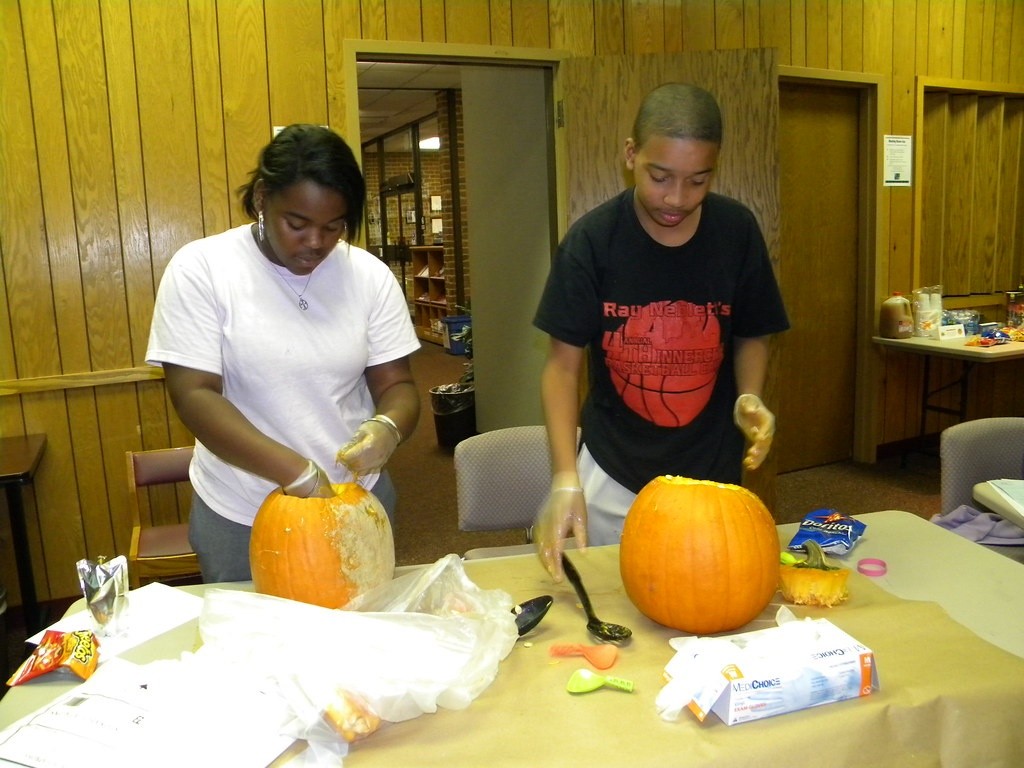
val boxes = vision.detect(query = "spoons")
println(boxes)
[559,553,631,640]
[510,595,554,636]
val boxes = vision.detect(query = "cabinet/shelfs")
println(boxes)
[410,244,446,345]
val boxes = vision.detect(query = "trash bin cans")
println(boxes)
[441,316,471,356]
[428,383,477,455]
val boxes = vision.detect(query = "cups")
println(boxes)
[915,292,942,338]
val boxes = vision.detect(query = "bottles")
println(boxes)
[879,292,914,339]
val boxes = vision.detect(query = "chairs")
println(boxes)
[939,417,1024,564]
[455,425,582,562]
[126,442,204,586]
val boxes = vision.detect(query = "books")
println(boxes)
[990,479,1024,519]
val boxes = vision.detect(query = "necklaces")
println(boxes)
[256,228,313,311]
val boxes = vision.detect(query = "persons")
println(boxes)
[533,83,791,582]
[143,123,422,583]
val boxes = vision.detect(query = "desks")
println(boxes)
[873,326,1024,466]
[0,509,1023,768]
[1,431,49,657]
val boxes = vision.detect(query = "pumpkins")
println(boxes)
[620,475,780,634]
[250,480,395,611]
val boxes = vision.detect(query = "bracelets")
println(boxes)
[361,418,401,445]
[281,457,320,498]
